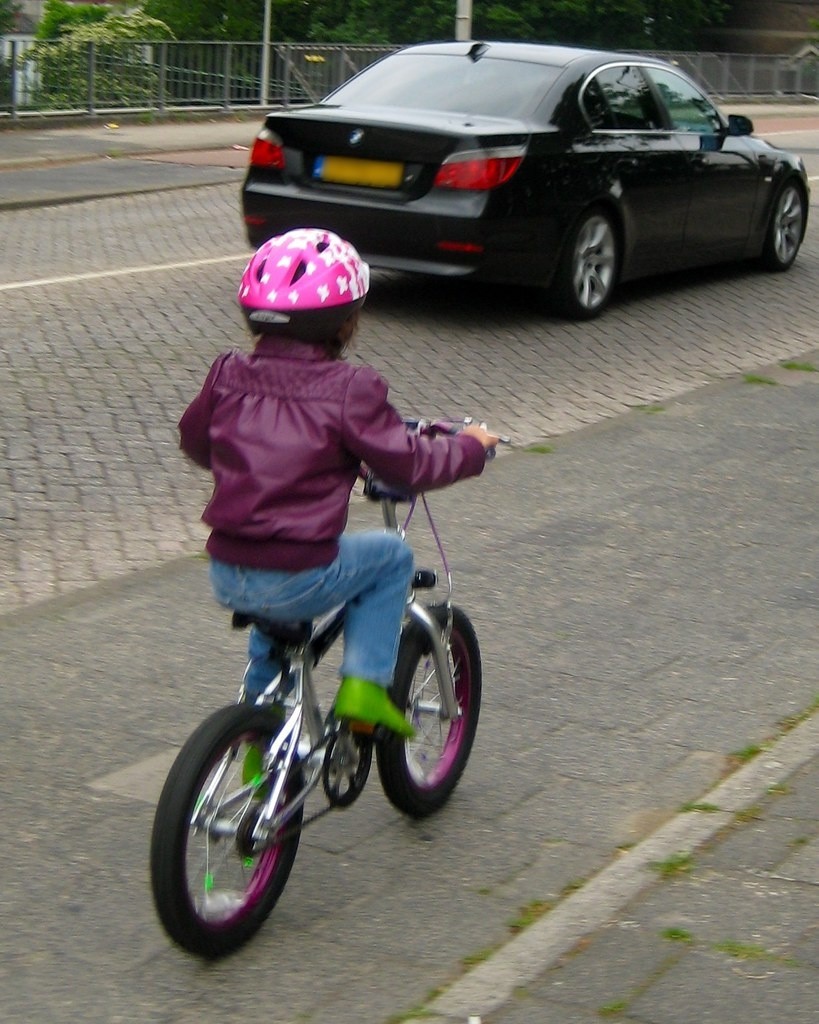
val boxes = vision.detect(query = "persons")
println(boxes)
[177,228,500,801]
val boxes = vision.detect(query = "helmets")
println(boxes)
[237,226,371,312]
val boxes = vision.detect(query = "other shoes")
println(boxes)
[334,675,418,739]
[242,705,287,801]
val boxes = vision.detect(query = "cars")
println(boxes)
[240,38,811,322]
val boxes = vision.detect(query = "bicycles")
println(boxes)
[149,416,513,959]
